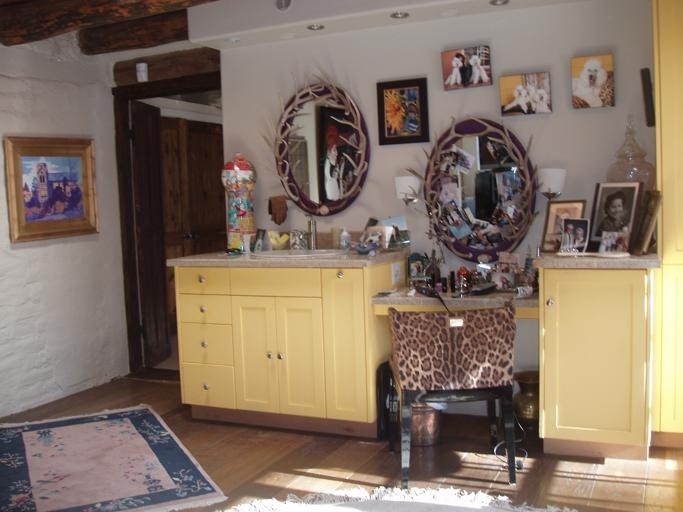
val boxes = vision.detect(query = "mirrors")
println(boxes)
[399,108,548,266]
[258,62,374,218]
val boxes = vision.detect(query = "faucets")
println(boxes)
[304,212,318,250]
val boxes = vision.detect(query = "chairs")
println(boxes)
[380,295,522,490]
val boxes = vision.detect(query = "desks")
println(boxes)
[371,286,539,324]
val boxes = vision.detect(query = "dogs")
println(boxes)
[469,55,490,85]
[503,86,551,113]
[572,60,608,108]
[444,57,464,86]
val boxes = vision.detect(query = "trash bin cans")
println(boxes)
[411,402,441,446]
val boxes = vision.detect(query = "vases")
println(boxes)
[219,148,258,253]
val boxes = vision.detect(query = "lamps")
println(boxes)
[392,175,421,209]
[535,166,569,201]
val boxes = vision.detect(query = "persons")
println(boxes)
[597,189,631,240]
[563,223,574,246]
[472,187,525,246]
[574,226,587,245]
[319,119,359,205]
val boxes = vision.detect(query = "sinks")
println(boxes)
[247,248,345,257]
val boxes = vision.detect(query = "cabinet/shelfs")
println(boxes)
[533,264,654,460]
[172,260,234,417]
[646,2,683,456]
[318,253,408,427]
[227,261,326,428]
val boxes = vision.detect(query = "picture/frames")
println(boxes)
[308,101,359,204]
[631,187,662,255]
[1,130,101,247]
[538,198,587,254]
[373,76,430,150]
[587,180,641,252]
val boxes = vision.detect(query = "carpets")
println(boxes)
[212,482,581,511]
[0,399,228,512]
